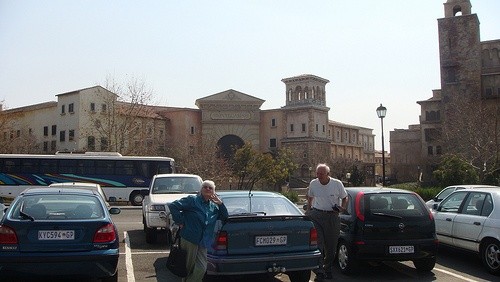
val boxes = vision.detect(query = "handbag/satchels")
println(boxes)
[167,229,187,277]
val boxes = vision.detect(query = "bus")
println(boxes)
[0,151,175,206]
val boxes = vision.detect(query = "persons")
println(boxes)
[304,162,349,282]
[168,179,230,282]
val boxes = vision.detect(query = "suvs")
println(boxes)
[140,174,205,244]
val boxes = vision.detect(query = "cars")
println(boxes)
[303,187,439,276]
[205,189,321,282]
[406,185,498,210]
[0,187,121,282]
[36,182,121,215]
[429,187,500,276]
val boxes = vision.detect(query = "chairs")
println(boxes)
[183,184,194,191]
[474,197,489,214]
[158,184,168,190]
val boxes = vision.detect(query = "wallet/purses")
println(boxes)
[334,204,341,212]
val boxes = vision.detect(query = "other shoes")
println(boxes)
[314,274,323,282]
[326,271,332,279]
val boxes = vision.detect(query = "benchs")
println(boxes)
[370,198,409,209]
[29,203,93,218]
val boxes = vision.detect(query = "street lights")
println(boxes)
[376,103,387,188]
